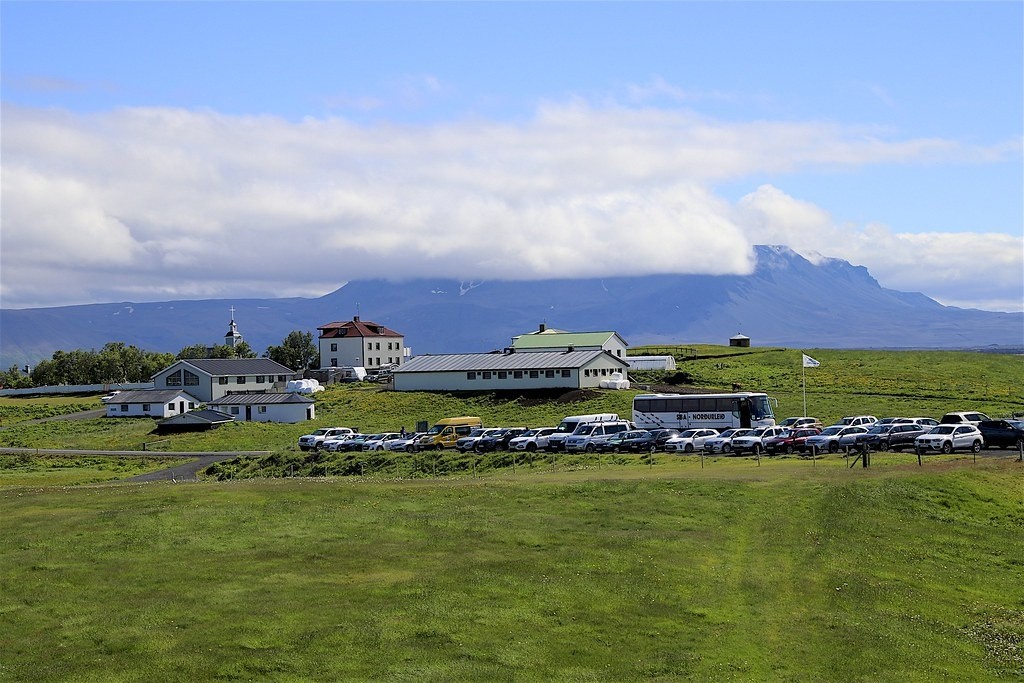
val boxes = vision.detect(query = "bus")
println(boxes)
[731,426,792,453]
[631,390,778,434]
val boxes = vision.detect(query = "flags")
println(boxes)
[803,354,821,369]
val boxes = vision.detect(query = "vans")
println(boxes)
[546,412,618,453]
[564,420,630,454]
[942,411,996,430]
[362,370,388,382]
[415,416,484,451]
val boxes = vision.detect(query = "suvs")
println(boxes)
[914,424,985,453]
[455,425,563,454]
[803,425,872,454]
[298,427,354,452]
[776,416,823,431]
[823,413,940,451]
[976,416,1024,451]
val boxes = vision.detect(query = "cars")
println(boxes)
[338,433,377,452]
[101,391,122,403]
[764,428,820,456]
[389,429,439,452]
[361,432,404,452]
[665,427,721,454]
[703,427,753,453]
[601,429,650,454]
[625,427,680,454]
[321,431,363,454]
